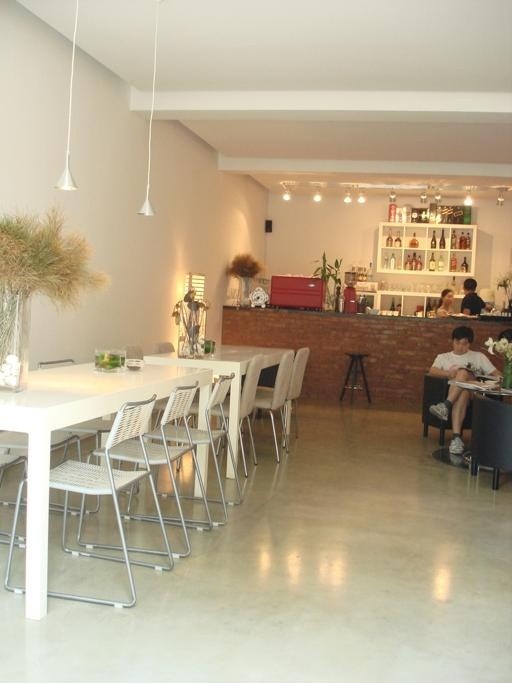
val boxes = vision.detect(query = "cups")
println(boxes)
[205,339,215,357]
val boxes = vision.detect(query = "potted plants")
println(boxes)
[494,272,511,312]
[0,208,108,390]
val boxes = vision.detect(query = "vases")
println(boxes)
[502,360,512,388]
[237,275,253,307]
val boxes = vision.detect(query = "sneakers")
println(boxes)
[431,402,449,421]
[449,436,465,455]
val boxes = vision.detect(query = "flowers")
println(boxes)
[484,338,512,361]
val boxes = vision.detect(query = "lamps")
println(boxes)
[136,1,158,217]
[57,0,78,192]
[419,188,428,204]
[495,188,507,208]
[185,272,204,304]
[432,186,442,203]
[387,187,398,203]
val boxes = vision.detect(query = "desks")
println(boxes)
[446,379,511,462]
[144,342,295,480]
[0,358,212,618]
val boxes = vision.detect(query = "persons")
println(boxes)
[428,325,507,455]
[460,278,493,315]
[434,288,454,319]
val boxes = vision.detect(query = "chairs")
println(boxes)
[422,372,473,446]
[471,391,512,489]
[0,430,85,537]
[142,372,242,527]
[4,396,173,608]
[78,382,213,556]
[187,355,258,477]
[35,360,138,514]
[229,353,293,463]
[255,346,309,447]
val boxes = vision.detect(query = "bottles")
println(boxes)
[461,257,468,272]
[416,256,422,270]
[430,230,436,248]
[386,230,392,247]
[405,255,412,270]
[352,261,373,283]
[451,231,456,249]
[438,255,444,271]
[440,229,445,249]
[384,257,388,269]
[429,253,436,271]
[409,233,418,248]
[391,298,395,310]
[390,254,396,270]
[459,232,466,249]
[466,233,470,250]
[451,254,457,272]
[451,277,456,290]
[395,231,401,247]
[412,253,416,270]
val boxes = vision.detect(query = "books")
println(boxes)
[457,367,497,381]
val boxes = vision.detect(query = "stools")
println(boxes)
[340,351,373,405]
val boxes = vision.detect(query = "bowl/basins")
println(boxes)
[127,360,142,371]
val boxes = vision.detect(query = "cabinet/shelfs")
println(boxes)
[376,291,465,318]
[376,221,478,277]
